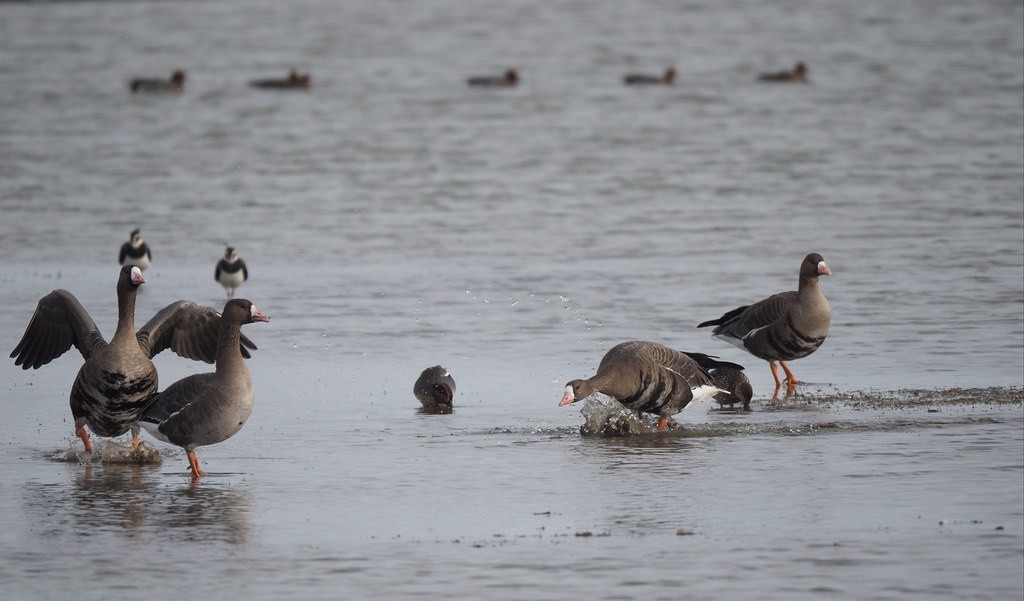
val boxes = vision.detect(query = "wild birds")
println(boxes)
[623,64,678,86]
[215,246,247,302]
[119,228,152,273]
[696,252,833,399]
[10,265,270,479]
[759,61,810,81]
[682,351,752,412]
[467,64,519,87]
[122,57,313,99]
[558,341,715,431]
[413,365,457,411]
